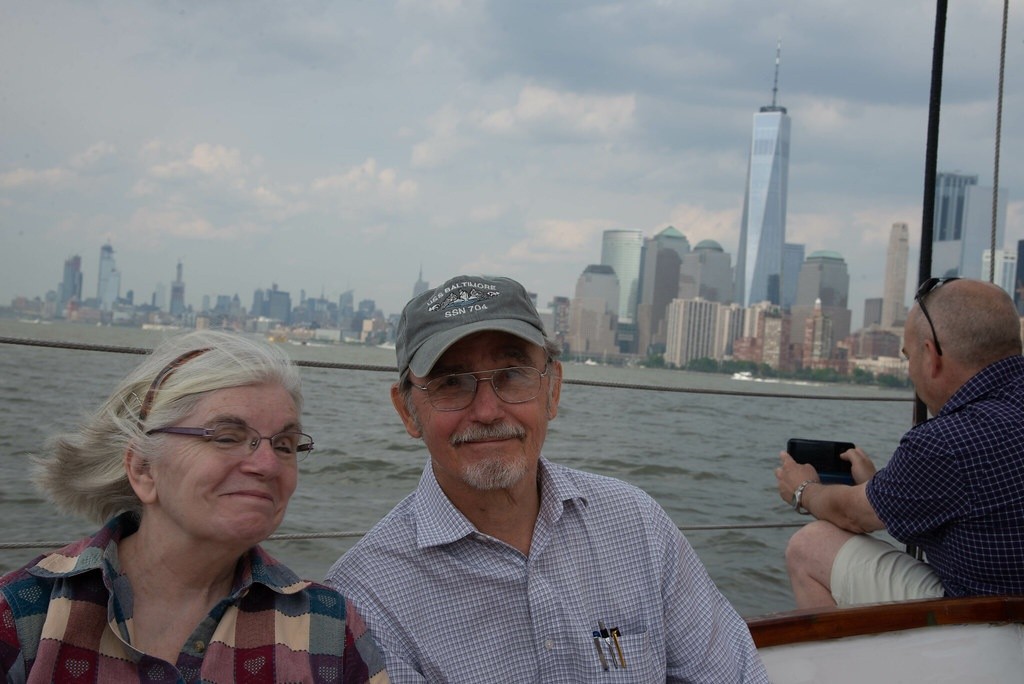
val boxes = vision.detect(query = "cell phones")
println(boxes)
[788,439,855,477]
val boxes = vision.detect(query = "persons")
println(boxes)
[772,274,1024,603]
[0,327,388,684]
[323,275,769,684]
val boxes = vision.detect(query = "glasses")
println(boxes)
[915,277,963,357]
[409,363,548,411]
[145,423,314,461]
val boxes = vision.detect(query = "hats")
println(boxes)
[395,275,553,378]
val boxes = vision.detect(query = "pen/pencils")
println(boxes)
[609,627,626,668]
[598,620,619,669]
[592,631,609,671]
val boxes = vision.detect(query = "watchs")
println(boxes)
[791,479,812,515]
